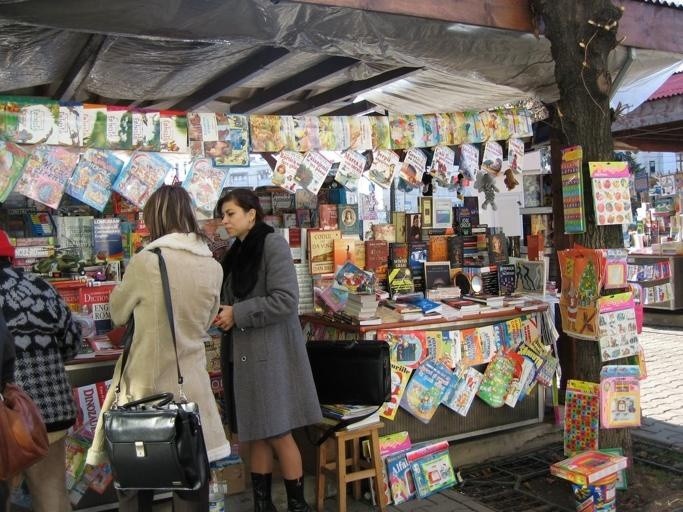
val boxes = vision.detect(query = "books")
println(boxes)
[1,190,150,430]
[622,171,682,313]
[254,180,556,430]
[1,93,534,217]
[194,217,238,417]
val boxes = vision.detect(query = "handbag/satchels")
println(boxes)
[306,340,391,403]
[103,392,211,491]
[0,383,49,481]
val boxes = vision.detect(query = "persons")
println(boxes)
[84,184,231,510]
[0,232,82,512]
[214,189,324,511]
[0,315,49,510]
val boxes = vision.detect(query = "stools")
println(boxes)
[113,477,207,511]
[316,414,388,511]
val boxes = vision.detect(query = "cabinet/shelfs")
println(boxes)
[627,254,682,310]
[300,299,552,453]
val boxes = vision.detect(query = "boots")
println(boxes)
[251,474,276,512]
[283,476,311,511]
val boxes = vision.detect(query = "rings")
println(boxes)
[217,320,220,325]
